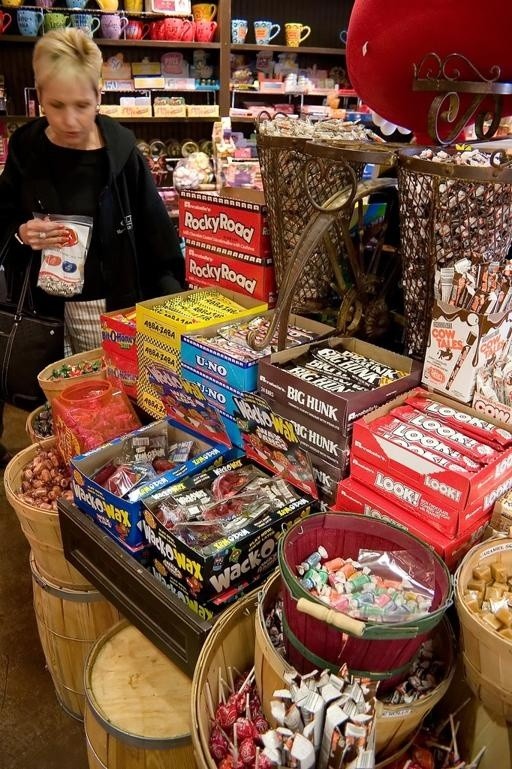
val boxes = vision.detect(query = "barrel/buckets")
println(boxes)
[4,344,512,769]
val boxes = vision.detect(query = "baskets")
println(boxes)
[255,131,512,359]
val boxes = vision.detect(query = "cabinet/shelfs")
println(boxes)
[1,38,365,222]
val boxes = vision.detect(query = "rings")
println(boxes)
[39,232,46,239]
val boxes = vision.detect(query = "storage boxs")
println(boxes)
[176,184,278,311]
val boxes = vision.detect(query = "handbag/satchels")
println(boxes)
[0,303,64,413]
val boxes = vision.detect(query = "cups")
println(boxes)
[70,13,101,36]
[101,15,129,39]
[192,3,217,22]
[2,0,155,13]
[0,13,12,34]
[254,20,281,44]
[45,12,71,32]
[231,19,248,44]
[125,20,150,40]
[150,17,218,43]
[15,10,45,37]
[284,22,311,47]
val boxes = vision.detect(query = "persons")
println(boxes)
[0,28,189,360]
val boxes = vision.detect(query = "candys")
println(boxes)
[14,117,512,769]
